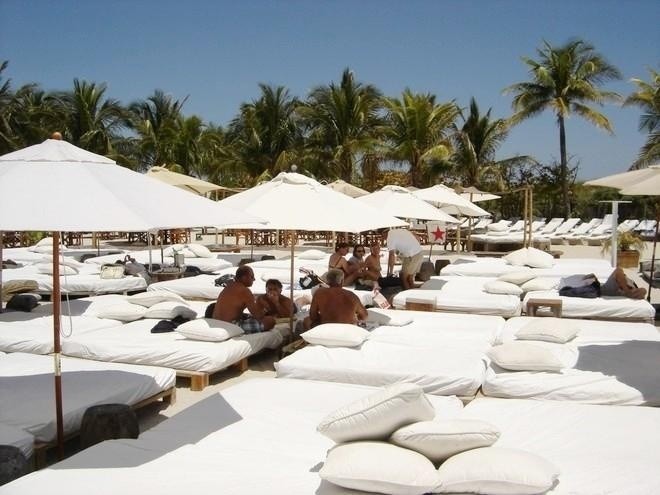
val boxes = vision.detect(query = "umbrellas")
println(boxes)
[207,172,409,348]
[0,131,270,464]
[581,165,660,301]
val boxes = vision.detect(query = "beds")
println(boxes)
[0,237,660,493]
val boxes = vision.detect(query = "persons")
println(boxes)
[350,244,379,290]
[366,244,385,281]
[257,279,297,318]
[378,227,423,291]
[210,263,279,332]
[302,269,369,331]
[330,244,366,286]
[601,268,646,299]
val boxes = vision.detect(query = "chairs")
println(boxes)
[60,222,389,248]
[412,217,655,251]
